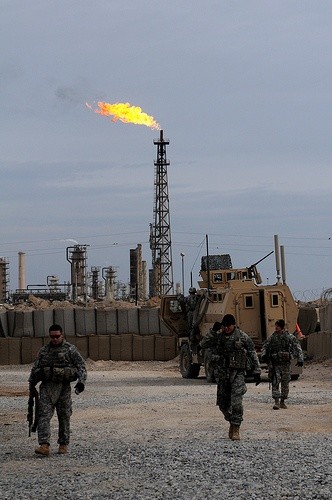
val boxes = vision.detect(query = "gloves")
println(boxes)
[254,375,261,386]
[74,382,84,395]
[29,388,38,397]
[212,321,222,331]
[295,362,303,367]
[262,355,270,364]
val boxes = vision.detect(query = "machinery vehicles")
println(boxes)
[159,234,304,379]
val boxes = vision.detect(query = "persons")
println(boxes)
[200,313,261,440]
[262,319,304,410]
[28,324,87,456]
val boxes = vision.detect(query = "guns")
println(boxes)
[267,362,271,388]
[27,387,38,436]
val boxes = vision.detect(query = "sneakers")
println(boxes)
[35,443,49,456]
[58,444,67,453]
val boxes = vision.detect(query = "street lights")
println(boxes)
[180,253,185,297]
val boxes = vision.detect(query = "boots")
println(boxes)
[273,398,280,409]
[229,424,233,438]
[232,424,240,441]
[280,398,287,409]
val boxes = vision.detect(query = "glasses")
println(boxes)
[49,334,62,339]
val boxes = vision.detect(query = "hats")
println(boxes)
[221,314,235,325]
[274,319,285,330]
